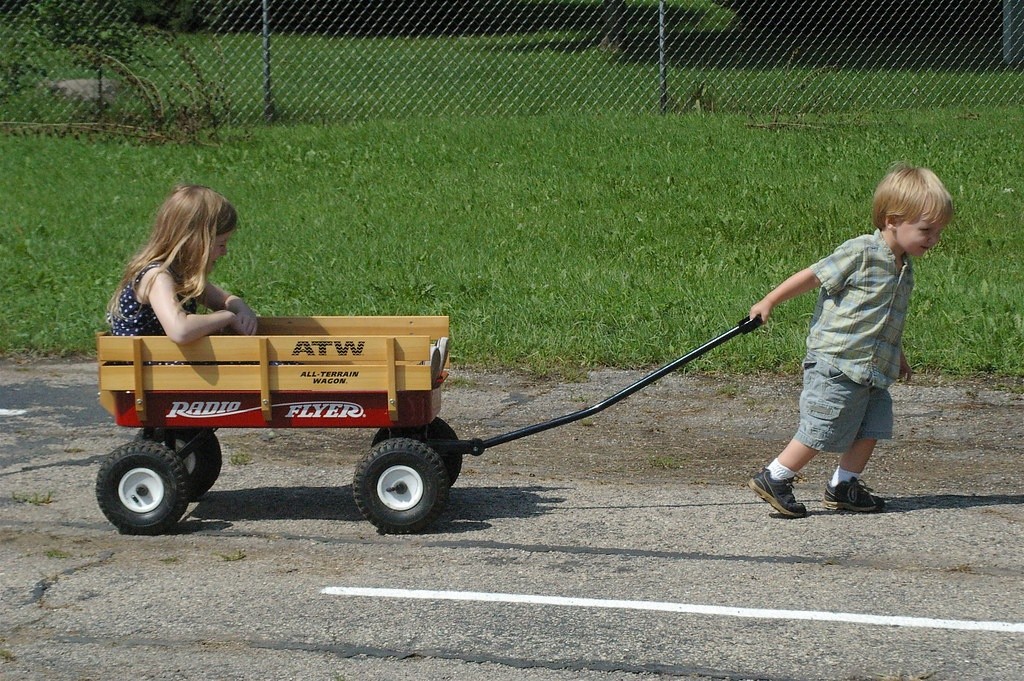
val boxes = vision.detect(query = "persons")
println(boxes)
[107,184,301,365]
[747,161,955,516]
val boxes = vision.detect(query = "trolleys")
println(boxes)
[97,304,768,534]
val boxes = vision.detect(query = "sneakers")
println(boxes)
[824,477,884,512]
[748,467,806,517]
